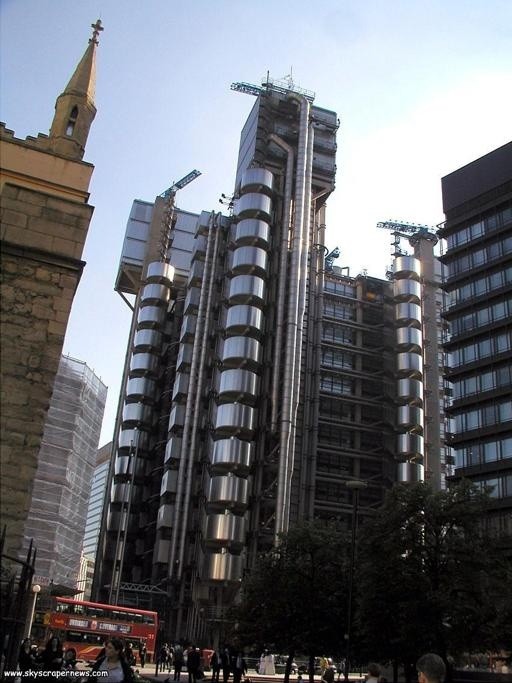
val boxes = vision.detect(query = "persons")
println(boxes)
[361,648,512,683]
[0,593,352,683]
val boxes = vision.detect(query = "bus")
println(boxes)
[31,593,158,665]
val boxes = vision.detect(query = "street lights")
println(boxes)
[343,476,368,681]
[27,584,42,638]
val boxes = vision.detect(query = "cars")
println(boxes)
[302,656,337,674]
[255,654,298,674]
